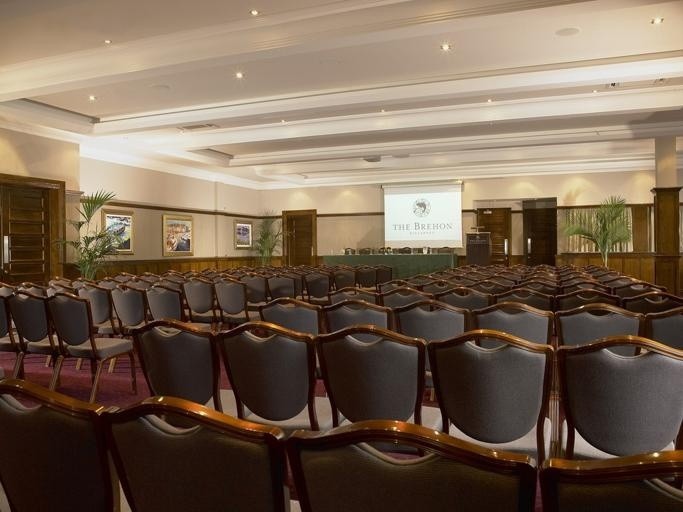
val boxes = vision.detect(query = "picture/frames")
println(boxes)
[163,214,195,256]
[100,207,134,256]
[234,218,253,250]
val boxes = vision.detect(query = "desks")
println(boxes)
[466,232,492,264]
[323,254,457,278]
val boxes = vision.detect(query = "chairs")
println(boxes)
[2,377,120,512]
[560,266,584,272]
[422,280,462,293]
[519,276,560,286]
[493,264,503,270]
[507,268,526,275]
[290,419,540,512]
[286,272,307,299]
[486,266,497,272]
[104,397,290,511]
[72,278,94,288]
[530,268,556,274]
[590,268,607,275]
[380,286,433,311]
[557,336,682,462]
[481,275,518,285]
[45,283,77,297]
[562,276,598,284]
[258,298,322,337]
[377,263,393,281]
[241,263,285,274]
[286,271,308,300]
[329,286,379,305]
[112,284,150,333]
[466,270,489,280]
[324,298,392,333]
[534,264,557,270]
[588,266,600,271]
[647,307,682,350]
[511,264,528,271]
[471,264,480,269]
[595,271,618,281]
[587,265,595,269]
[557,303,644,356]
[624,292,682,312]
[435,287,491,309]
[160,273,185,285]
[162,270,184,276]
[216,320,346,436]
[541,450,683,512]
[450,267,464,274]
[184,279,219,320]
[393,299,469,402]
[269,275,295,299]
[186,266,240,278]
[153,278,184,310]
[316,324,441,436]
[431,271,451,278]
[470,303,554,348]
[356,263,376,287]
[97,279,120,289]
[377,280,420,292]
[605,277,633,286]
[131,320,250,423]
[561,268,586,275]
[496,270,521,280]
[527,271,559,280]
[406,274,438,283]
[286,264,356,271]
[460,266,471,270]
[560,264,578,268]
[104,275,113,281]
[146,284,189,322]
[448,275,478,285]
[115,272,134,283]
[241,273,270,303]
[479,267,494,276]
[305,273,332,300]
[427,330,552,465]
[345,247,454,254]
[465,280,511,294]
[138,272,159,282]
[563,281,612,294]
[0,281,14,297]
[512,264,531,269]
[513,281,560,294]
[614,281,667,300]
[554,290,621,307]
[494,288,553,310]
[16,282,47,296]
[49,276,71,286]
[562,272,591,280]
[79,284,117,332]
[124,277,150,290]
[214,278,249,322]
[45,291,139,405]
[335,270,354,290]
